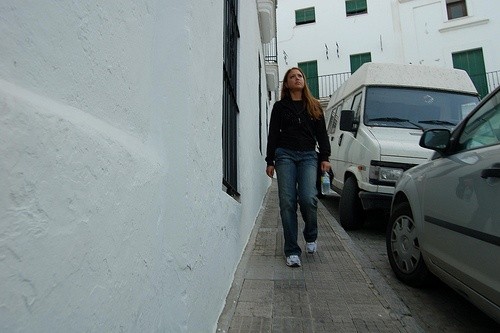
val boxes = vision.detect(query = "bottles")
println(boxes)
[321,171,330,194]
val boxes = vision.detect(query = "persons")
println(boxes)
[455,163,500,237]
[265,67,331,268]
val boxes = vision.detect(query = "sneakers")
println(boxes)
[286,242,317,267]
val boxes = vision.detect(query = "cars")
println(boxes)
[385,85,500,326]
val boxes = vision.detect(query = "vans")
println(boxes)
[317,62,500,231]
